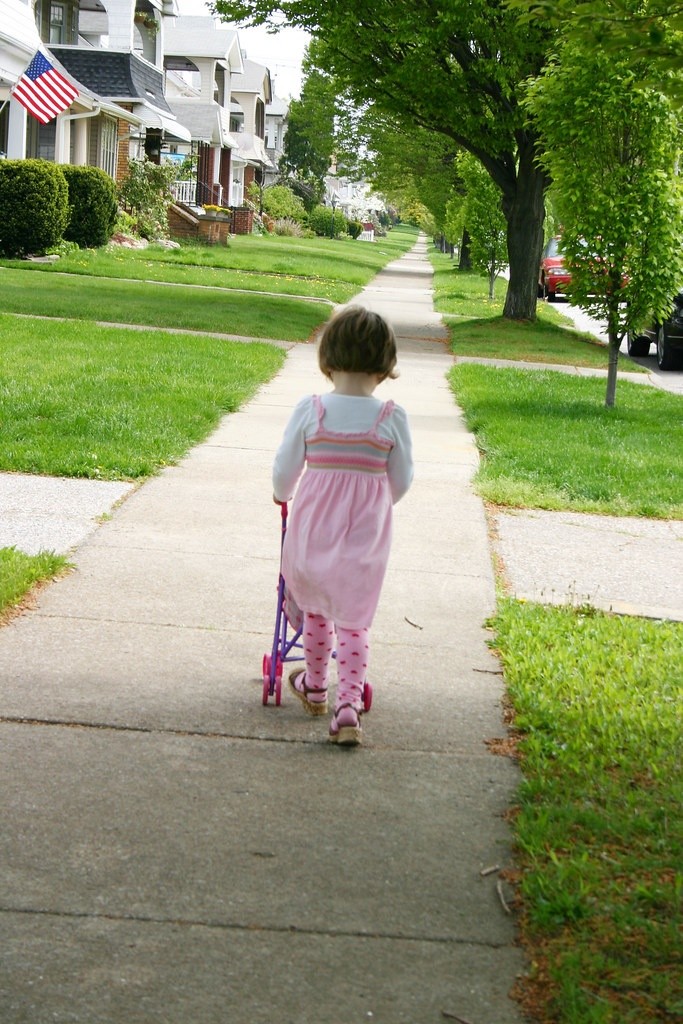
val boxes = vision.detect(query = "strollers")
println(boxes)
[261,498,372,714]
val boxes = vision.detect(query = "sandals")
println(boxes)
[288,669,328,717]
[329,703,362,745]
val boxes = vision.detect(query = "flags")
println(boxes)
[8,50,79,127]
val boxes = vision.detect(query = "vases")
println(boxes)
[143,18,160,40]
[217,212,228,218]
[134,11,148,24]
[206,211,217,217]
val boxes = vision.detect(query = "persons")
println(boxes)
[271,304,415,743]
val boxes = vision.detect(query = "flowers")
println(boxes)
[220,208,231,215]
[202,203,221,212]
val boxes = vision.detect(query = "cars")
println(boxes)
[626,287,683,371]
[537,234,631,304]
